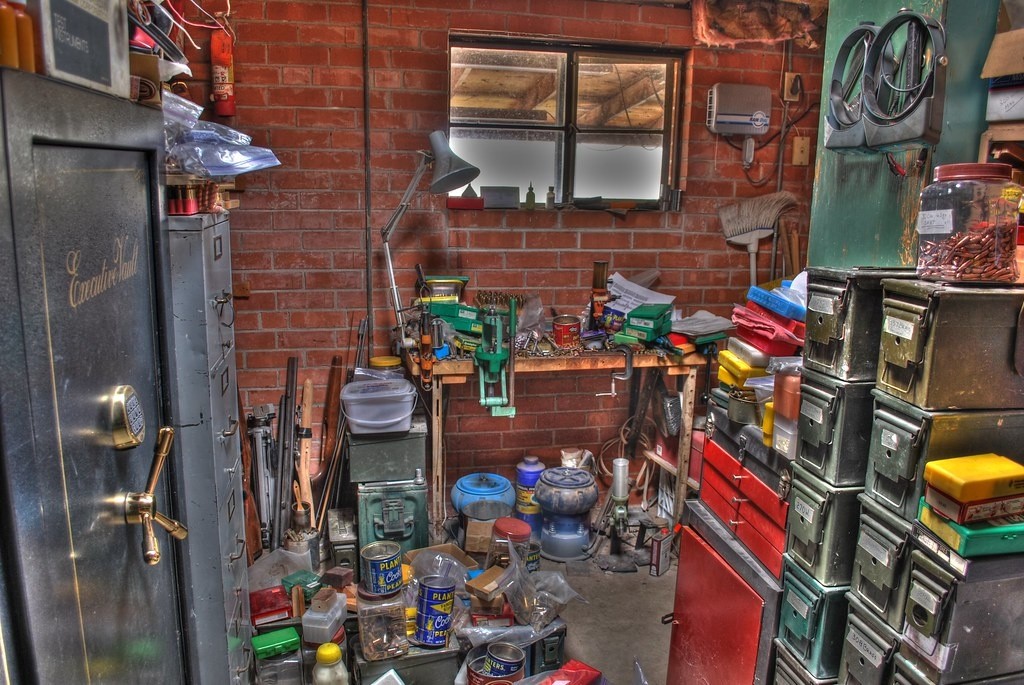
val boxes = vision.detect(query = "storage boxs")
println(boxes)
[708,26,1024,685]
[281,569,323,600]
[453,330,482,352]
[427,301,479,320]
[251,627,300,660]
[249,585,292,626]
[349,631,461,685]
[413,296,459,310]
[439,315,483,335]
[302,593,347,644]
[614,331,643,345]
[424,279,464,300]
[530,615,567,676]
[447,197,484,211]
[404,542,478,571]
[626,303,672,329]
[345,403,427,482]
[340,379,416,433]
[623,320,673,342]
[466,521,495,552]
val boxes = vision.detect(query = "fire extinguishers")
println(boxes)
[205,11,238,116]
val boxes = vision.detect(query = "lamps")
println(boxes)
[381,129,480,347]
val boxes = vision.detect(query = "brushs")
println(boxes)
[293,479,305,511]
[657,374,682,437]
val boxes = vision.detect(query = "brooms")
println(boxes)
[717,190,798,287]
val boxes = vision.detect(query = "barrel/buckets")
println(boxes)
[464,641,525,685]
[527,540,541,572]
[414,575,457,647]
[359,539,403,595]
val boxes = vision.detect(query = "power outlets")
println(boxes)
[783,71,800,102]
[792,136,810,166]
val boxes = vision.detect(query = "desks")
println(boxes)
[406,346,718,546]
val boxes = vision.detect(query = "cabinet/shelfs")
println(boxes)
[661,499,783,685]
[169,211,254,685]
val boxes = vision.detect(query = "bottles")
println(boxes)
[483,516,532,571]
[525,181,536,210]
[544,186,556,210]
[311,643,349,685]
[917,163,1024,283]
